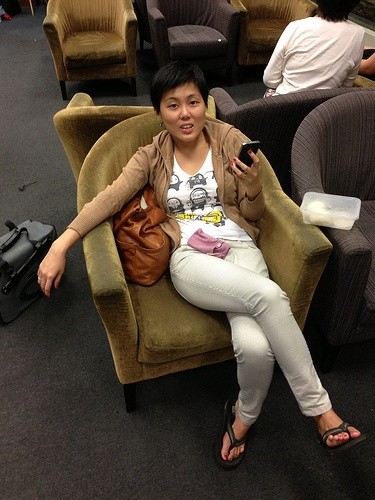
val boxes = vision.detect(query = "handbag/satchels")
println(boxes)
[113,186,169,286]
[0,220,58,324]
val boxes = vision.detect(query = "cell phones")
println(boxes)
[233,141,260,174]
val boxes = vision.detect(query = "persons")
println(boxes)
[263,0,366,98]
[37,61,369,466]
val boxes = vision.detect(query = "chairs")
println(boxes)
[41,0,375,412]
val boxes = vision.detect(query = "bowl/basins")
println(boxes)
[298,190,362,232]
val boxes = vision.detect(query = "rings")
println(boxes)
[38,277,41,280]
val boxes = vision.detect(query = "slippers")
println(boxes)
[215,399,247,468]
[319,426,367,452]
